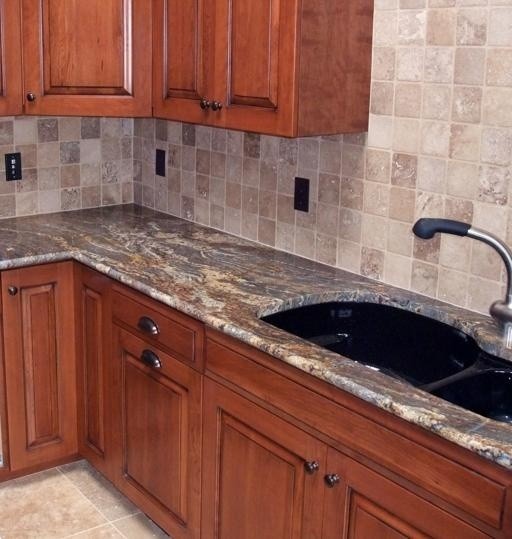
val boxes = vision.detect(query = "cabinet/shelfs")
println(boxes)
[0,261,81,483]
[0,0,153,118]
[203,334,512,539]
[153,0,374,139]
[81,264,202,539]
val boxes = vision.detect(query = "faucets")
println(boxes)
[411,217,512,305]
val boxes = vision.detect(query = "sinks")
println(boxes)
[427,368,512,427]
[259,300,475,388]
